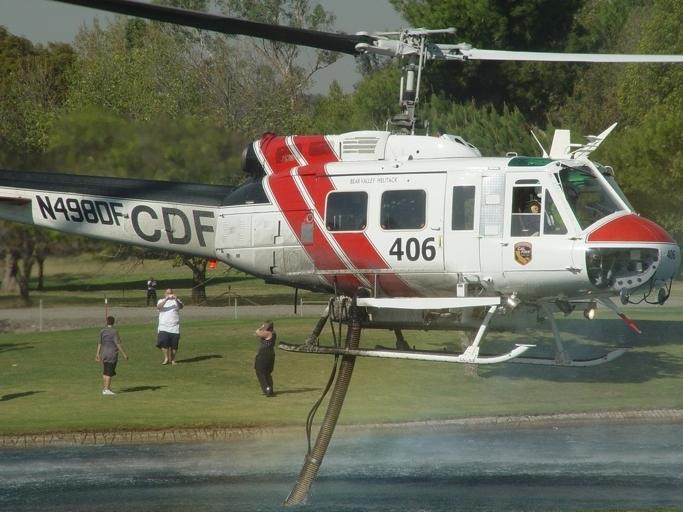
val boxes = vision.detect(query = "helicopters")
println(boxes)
[0,0,683,367]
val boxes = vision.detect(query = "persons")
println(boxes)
[146,277,158,307]
[95,316,127,395]
[157,288,184,366]
[254,320,277,397]
[521,193,554,235]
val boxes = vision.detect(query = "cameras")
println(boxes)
[169,295,174,299]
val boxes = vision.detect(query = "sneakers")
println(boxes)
[162,359,177,366]
[266,386,272,396]
[102,389,115,395]
